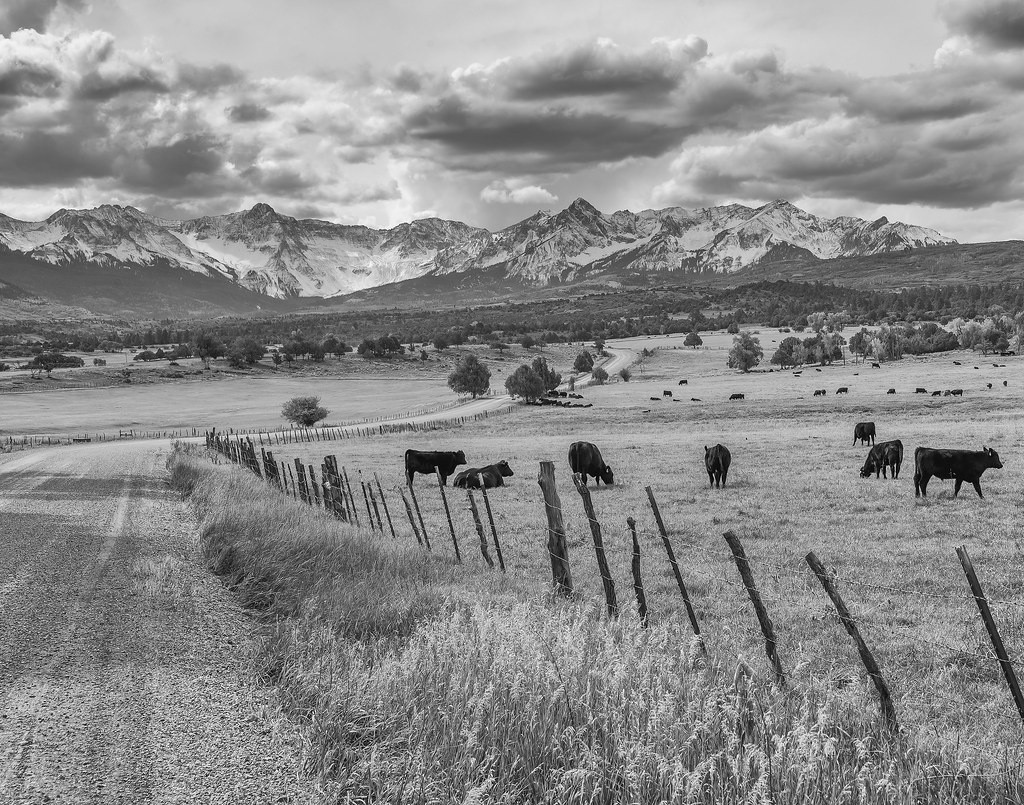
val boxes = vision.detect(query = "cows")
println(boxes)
[986,383,993,389]
[729,393,744,401]
[678,379,687,385]
[812,389,826,397]
[526,391,593,408]
[663,390,673,396]
[871,362,881,369]
[887,388,896,395]
[915,387,963,396]
[404,449,514,490]
[568,440,614,488]
[673,399,680,401]
[690,397,702,402]
[1003,380,1008,387]
[836,387,848,394]
[852,421,1003,498]
[953,349,1015,369]
[792,368,822,378]
[650,397,661,400]
[853,373,859,375]
[704,444,732,489]
